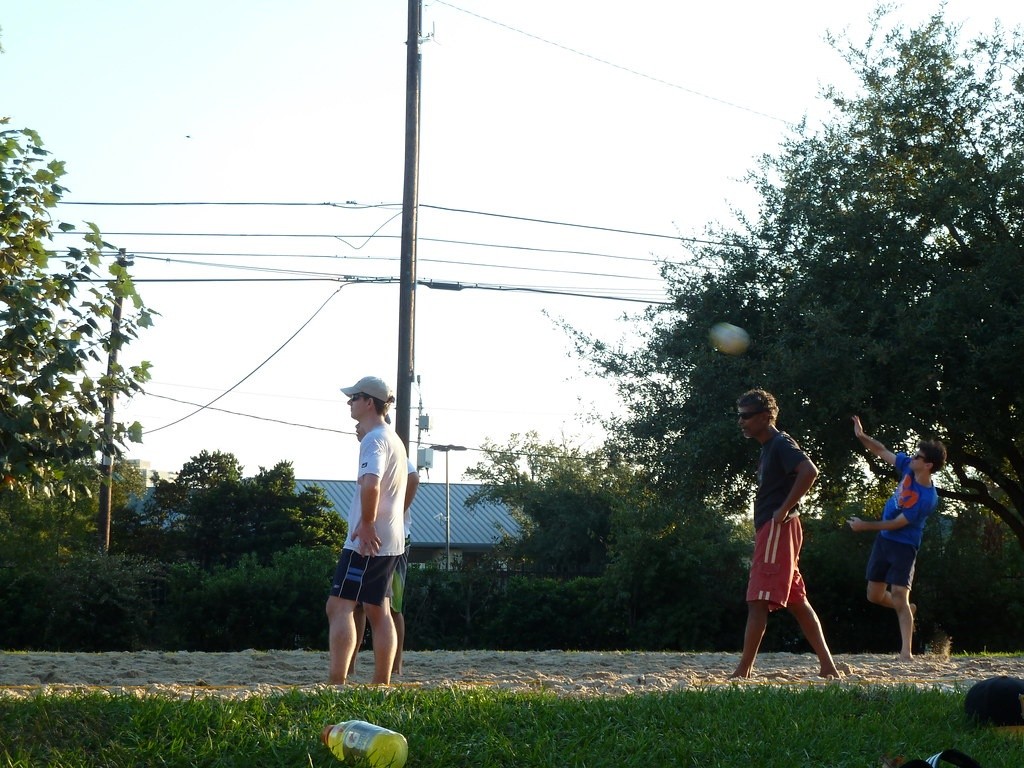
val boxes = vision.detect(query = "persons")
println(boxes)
[846,414,946,661]
[322,375,421,690]
[727,390,837,683]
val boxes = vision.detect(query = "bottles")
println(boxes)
[321,719,408,768]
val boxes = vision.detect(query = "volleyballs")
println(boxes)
[709,320,748,355]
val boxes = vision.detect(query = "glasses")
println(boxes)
[737,410,764,419]
[915,451,927,459]
[351,394,376,403]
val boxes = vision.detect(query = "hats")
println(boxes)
[340,376,389,403]
